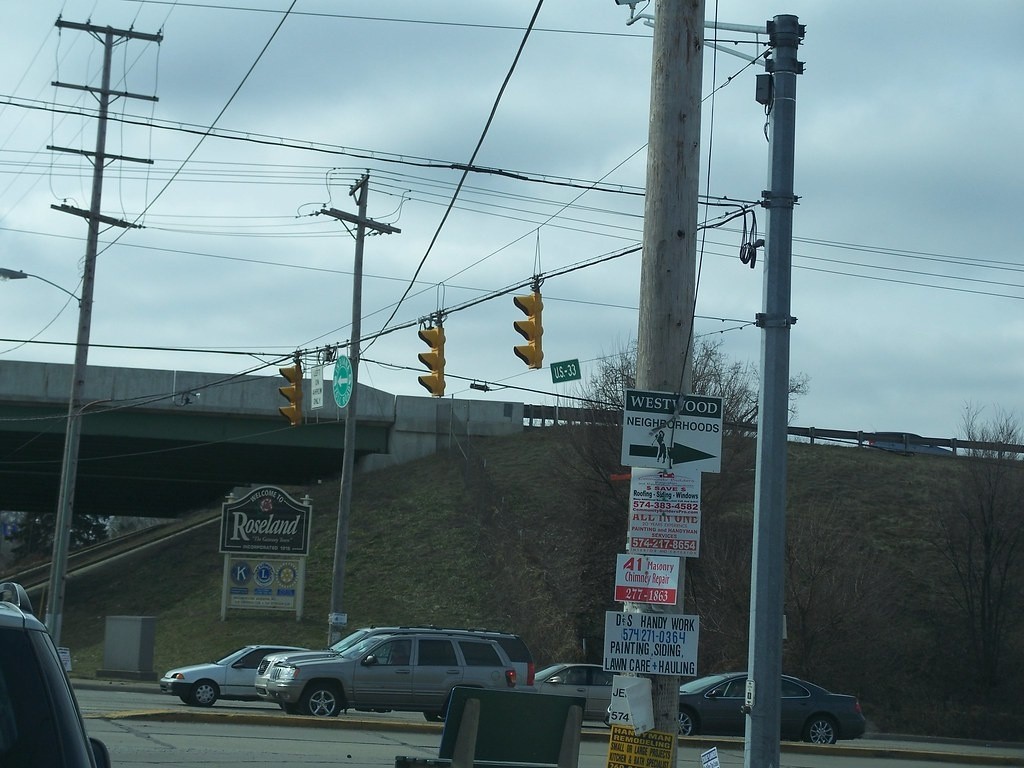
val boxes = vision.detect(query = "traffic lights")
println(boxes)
[416,327,447,396]
[278,364,303,427]
[512,293,545,370]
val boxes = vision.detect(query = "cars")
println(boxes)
[160,643,311,707]
[603,668,870,746]
[534,661,623,720]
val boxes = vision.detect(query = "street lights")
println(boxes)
[0,266,96,647]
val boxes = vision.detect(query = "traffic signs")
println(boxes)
[617,388,727,474]
[330,355,353,408]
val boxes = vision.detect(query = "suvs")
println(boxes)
[0,580,113,768]
[254,621,537,714]
[267,632,520,721]
